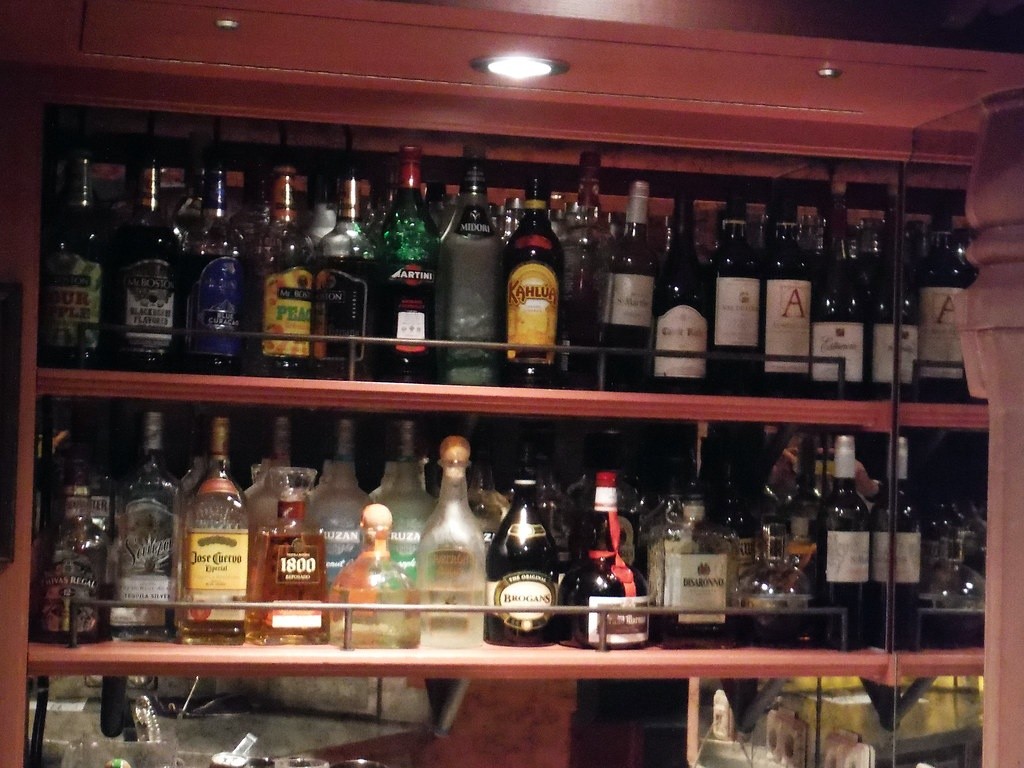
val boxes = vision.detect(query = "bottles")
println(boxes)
[36,411,962,650]
[40,146,974,402]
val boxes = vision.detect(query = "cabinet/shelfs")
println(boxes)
[0,0,1024,768]
